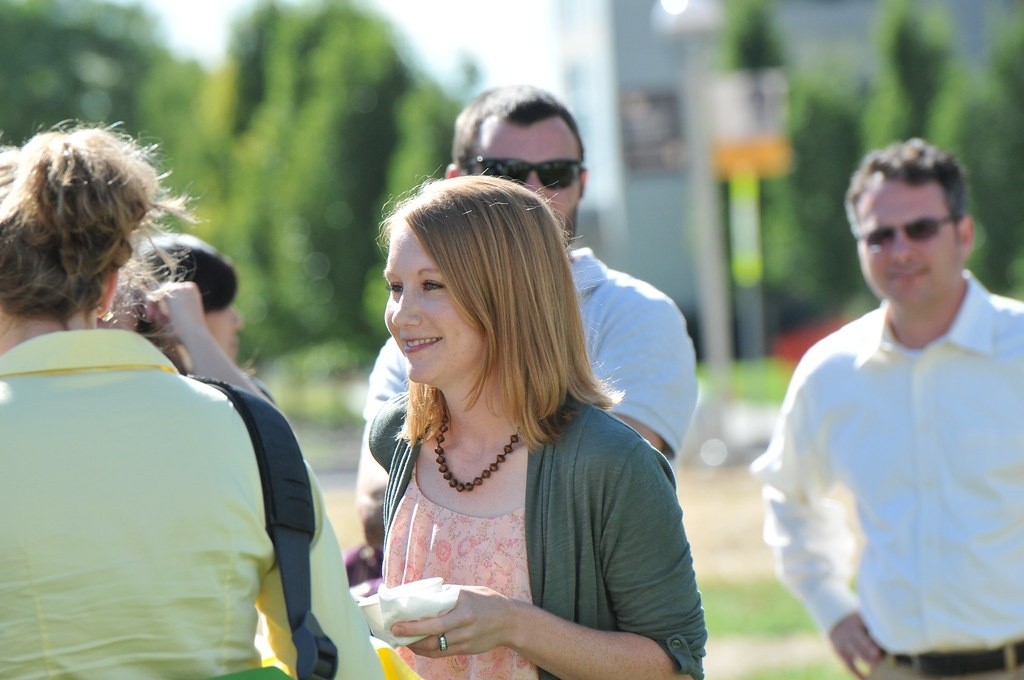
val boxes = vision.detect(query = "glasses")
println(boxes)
[855,216,954,246]
[464,156,584,190]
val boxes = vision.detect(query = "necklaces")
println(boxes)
[435,406,523,493]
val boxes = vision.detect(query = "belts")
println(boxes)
[876,638,1023,679]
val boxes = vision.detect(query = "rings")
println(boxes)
[438,633,450,653]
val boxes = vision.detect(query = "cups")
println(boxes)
[357,576,444,648]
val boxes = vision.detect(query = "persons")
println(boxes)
[355,82,687,552]
[758,133,1023,680]
[114,235,244,388]
[0,124,307,680]
[99,259,272,432]
[355,172,705,679]
